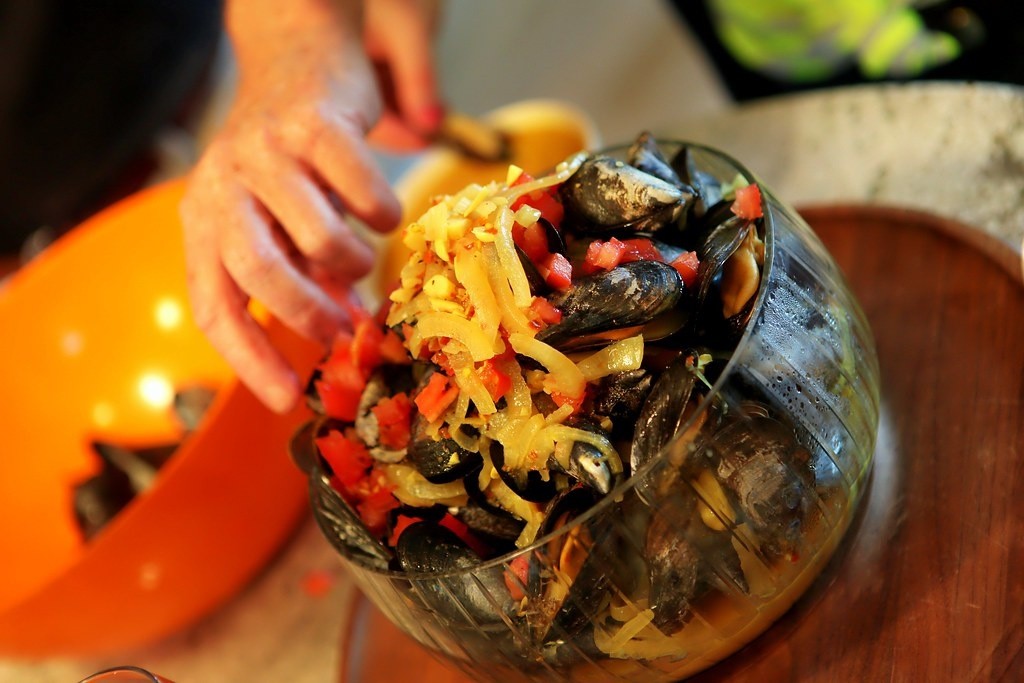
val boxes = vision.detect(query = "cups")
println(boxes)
[79,666,176,683]
[365,102,592,293]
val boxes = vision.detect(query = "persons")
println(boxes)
[361,0,1024,151]
[0,1,400,539]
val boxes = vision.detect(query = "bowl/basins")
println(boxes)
[312,141,879,683]
[1,177,314,657]
